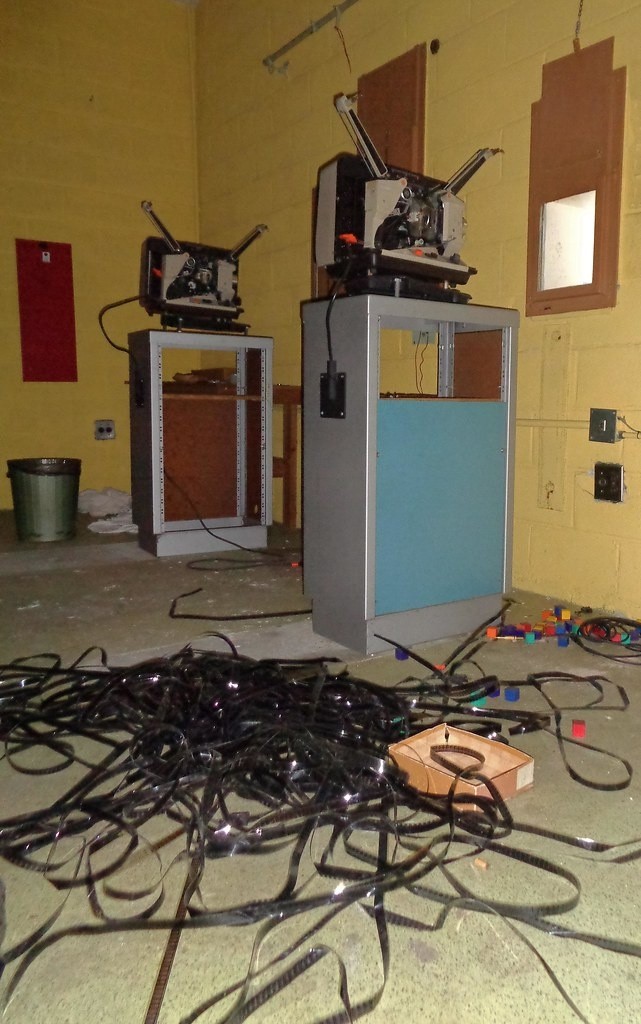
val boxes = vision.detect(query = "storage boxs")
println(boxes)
[386,724,535,815]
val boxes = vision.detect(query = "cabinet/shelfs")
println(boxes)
[127,327,301,559]
[299,294,520,654]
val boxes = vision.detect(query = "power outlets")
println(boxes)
[589,408,618,443]
[95,420,115,440]
[595,463,624,501]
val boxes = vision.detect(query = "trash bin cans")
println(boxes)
[7,457,82,543]
